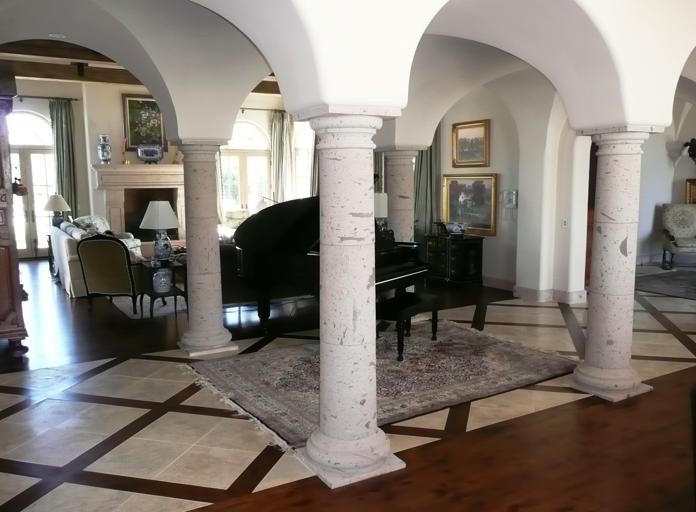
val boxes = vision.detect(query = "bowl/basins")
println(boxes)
[446,222,468,235]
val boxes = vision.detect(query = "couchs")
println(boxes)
[49,215,142,300]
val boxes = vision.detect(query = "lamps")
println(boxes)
[44,192,72,227]
[139,200,181,261]
[374,193,387,231]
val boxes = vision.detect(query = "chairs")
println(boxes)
[76,235,142,314]
[661,203,696,270]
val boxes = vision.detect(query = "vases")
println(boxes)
[98,135,112,165]
[153,268,171,292]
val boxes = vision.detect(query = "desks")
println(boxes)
[139,260,187,320]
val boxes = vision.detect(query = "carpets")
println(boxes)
[220,273,315,308]
[105,271,187,320]
[175,314,583,452]
[635,260,695,300]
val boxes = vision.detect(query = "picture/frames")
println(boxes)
[442,119,499,236]
[121,93,168,152]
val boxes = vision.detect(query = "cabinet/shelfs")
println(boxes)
[423,234,486,289]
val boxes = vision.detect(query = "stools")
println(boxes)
[376,292,439,361]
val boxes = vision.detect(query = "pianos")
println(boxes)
[234,196,439,361]
